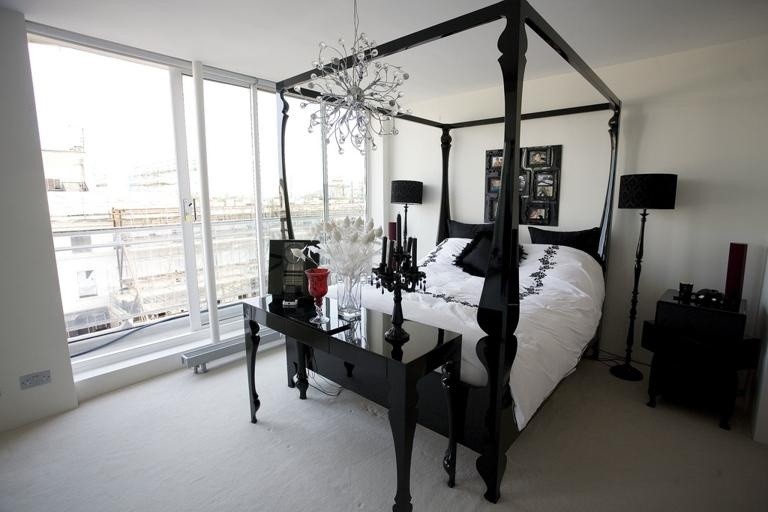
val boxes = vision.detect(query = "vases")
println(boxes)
[335,272,362,319]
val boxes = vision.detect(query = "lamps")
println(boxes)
[292,1,414,157]
[390,179,422,251]
[609,172,678,381]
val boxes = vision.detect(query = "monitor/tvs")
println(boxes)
[267,239,320,316]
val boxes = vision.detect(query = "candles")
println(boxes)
[380,214,417,272]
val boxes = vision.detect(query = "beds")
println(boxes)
[286,242,606,503]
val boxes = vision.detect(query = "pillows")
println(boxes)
[445,217,601,278]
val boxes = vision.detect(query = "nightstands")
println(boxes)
[646,288,748,430]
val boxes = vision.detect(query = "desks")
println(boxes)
[239,294,463,512]
